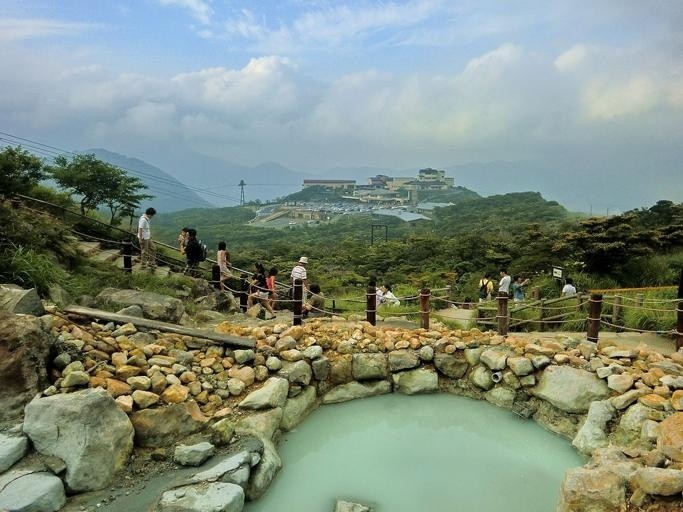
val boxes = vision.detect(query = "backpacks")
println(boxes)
[198,239,207,261]
[480,279,489,298]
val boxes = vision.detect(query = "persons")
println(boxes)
[478,268,531,304]
[178,228,206,278]
[248,257,325,320]
[216,241,233,291]
[376,283,401,310]
[137,207,157,270]
[560,277,576,298]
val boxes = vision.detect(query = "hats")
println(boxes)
[299,257,308,264]
